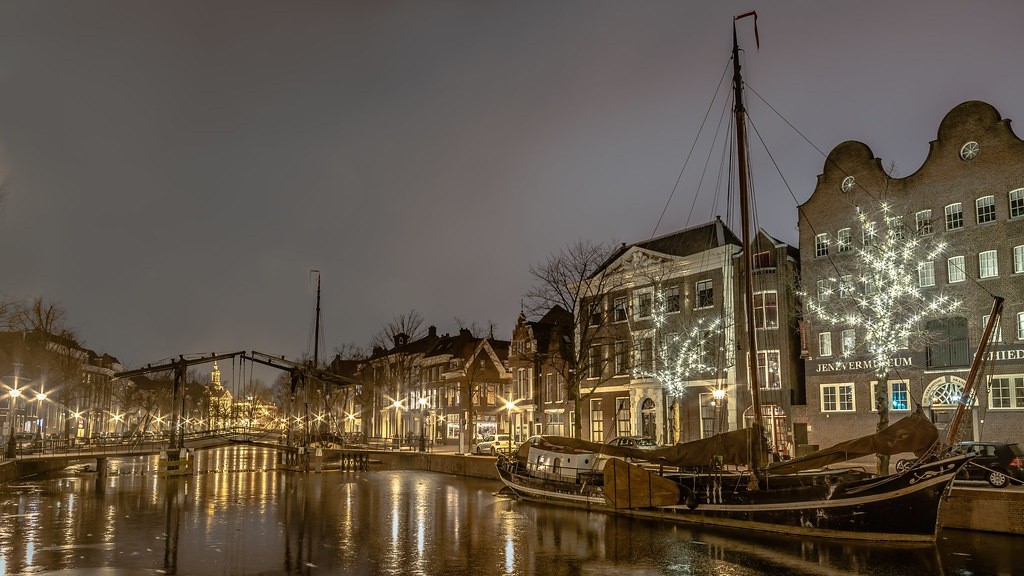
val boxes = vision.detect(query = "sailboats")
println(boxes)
[303,268,346,450]
[493,12,1007,546]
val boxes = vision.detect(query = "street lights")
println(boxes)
[33,393,47,453]
[349,414,353,433]
[418,398,426,451]
[506,401,514,456]
[7,389,21,457]
[393,400,400,449]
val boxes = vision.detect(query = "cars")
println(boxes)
[14,434,35,455]
[91,429,171,444]
[476,435,516,456]
[607,435,659,450]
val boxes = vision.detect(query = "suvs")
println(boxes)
[910,440,1024,488]
